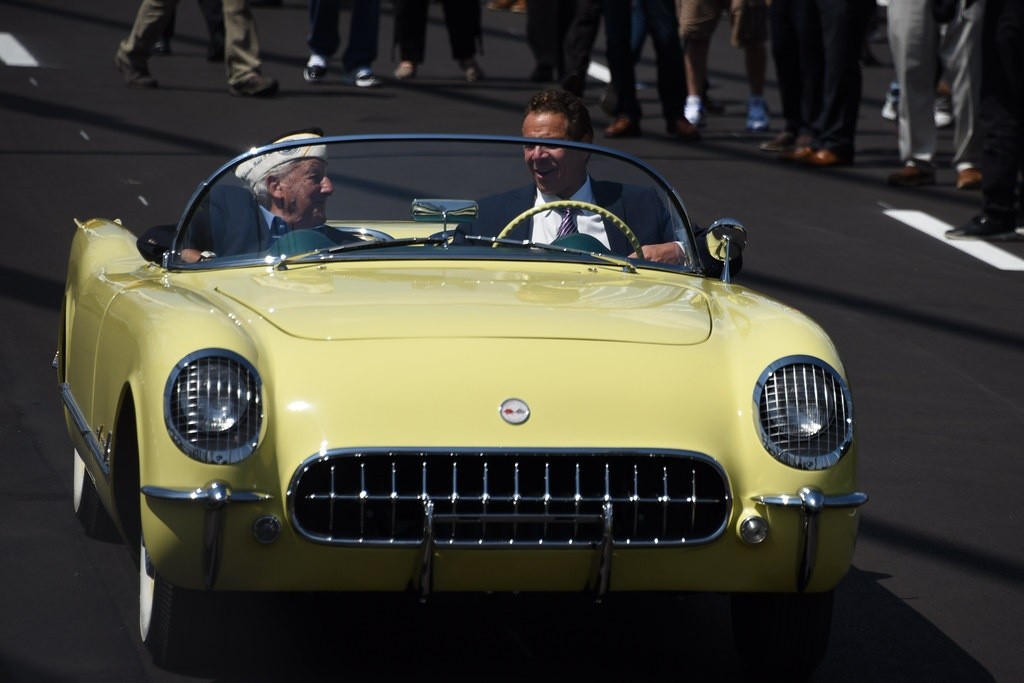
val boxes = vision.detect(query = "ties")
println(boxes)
[557,206,578,238]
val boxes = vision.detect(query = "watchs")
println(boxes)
[200,250,216,263]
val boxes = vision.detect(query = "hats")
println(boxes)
[234,127,330,187]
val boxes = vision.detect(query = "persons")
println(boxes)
[113,0,1023,239]
[452,88,741,278]
[182,132,332,257]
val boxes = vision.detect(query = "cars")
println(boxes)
[47,132,868,683]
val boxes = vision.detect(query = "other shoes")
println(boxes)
[530,61,553,82]
[510,0,528,13]
[206,47,226,63]
[354,63,384,87]
[155,39,172,56]
[115,54,158,89]
[892,158,1024,242]
[393,60,414,81]
[602,99,842,166]
[465,66,484,83]
[230,74,280,98]
[882,91,900,121]
[486,0,510,12]
[303,52,333,82]
[558,74,587,94]
[934,93,954,127]
